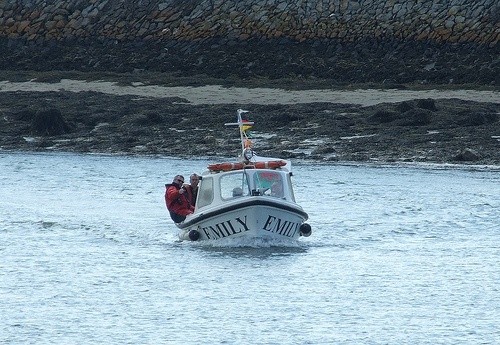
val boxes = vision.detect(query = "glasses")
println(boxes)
[176,178,184,183]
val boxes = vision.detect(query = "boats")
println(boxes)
[176,109,313,243]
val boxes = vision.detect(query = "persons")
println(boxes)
[181,173,206,214]
[231,185,244,199]
[164,175,195,224]
[269,181,287,201]
